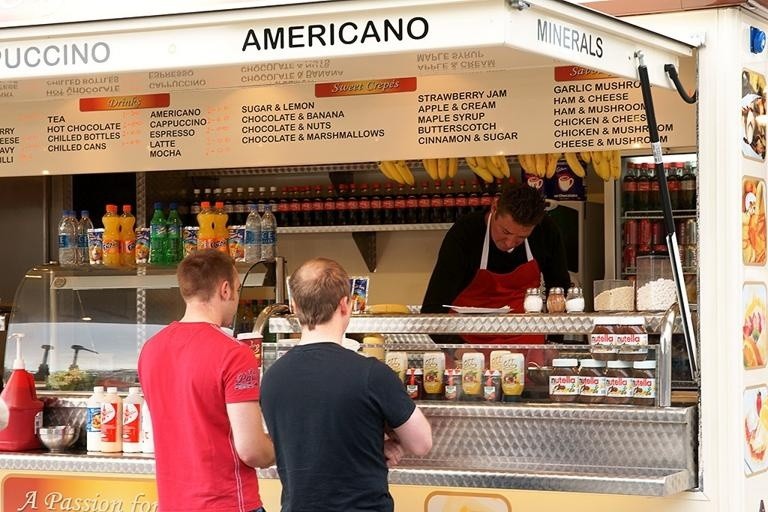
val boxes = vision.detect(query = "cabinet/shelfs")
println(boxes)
[267,303,698,424]
[0,257,284,400]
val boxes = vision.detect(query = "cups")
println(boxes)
[528,177,543,189]
[558,175,574,191]
[236,332,263,370]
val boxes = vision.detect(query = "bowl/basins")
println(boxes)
[39,425,81,454]
[528,367,554,385]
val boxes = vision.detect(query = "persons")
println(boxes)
[136,248,276,512]
[258,255,434,512]
[420,179,571,385]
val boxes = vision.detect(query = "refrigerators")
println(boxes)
[602,145,701,393]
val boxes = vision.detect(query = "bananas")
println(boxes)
[366,303,415,315]
[422,158,459,180]
[563,149,622,182]
[375,161,414,184]
[465,156,510,183]
[518,153,562,180]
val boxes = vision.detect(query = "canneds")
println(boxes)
[405,369,423,401]
[622,219,696,303]
[480,370,502,401]
[443,369,462,401]
[550,316,655,406]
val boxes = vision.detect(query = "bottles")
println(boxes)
[371,183,382,225]
[86,385,155,454]
[523,287,585,314]
[444,181,456,223]
[430,180,443,223]
[179,180,301,227]
[385,350,525,402]
[406,184,418,224]
[360,184,371,225]
[301,184,314,226]
[336,184,347,226]
[395,184,406,224]
[312,185,324,226]
[621,161,697,210]
[325,184,336,226]
[347,184,359,225]
[419,183,430,224]
[457,180,502,227]
[383,183,395,224]
[57,201,277,268]
